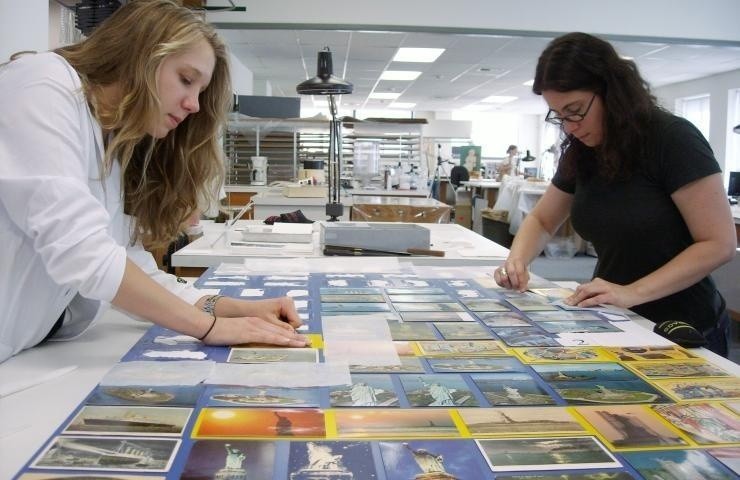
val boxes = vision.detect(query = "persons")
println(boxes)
[492,31,738,361]
[0,0,314,369]
[496,144,536,184]
[462,147,479,176]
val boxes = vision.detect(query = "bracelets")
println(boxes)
[197,310,216,342]
[202,293,226,317]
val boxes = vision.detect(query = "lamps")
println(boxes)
[297,46,353,221]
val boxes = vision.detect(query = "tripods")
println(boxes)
[424,147,456,198]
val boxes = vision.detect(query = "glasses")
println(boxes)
[544,94,599,126]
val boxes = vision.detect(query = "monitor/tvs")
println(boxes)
[524,168,537,179]
[727,172,740,196]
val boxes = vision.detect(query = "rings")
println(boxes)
[499,266,507,276]
